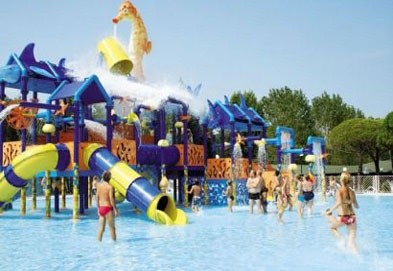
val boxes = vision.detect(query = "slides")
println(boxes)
[83,143,187,226]
[0,142,70,215]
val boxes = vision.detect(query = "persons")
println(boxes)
[223,180,234,211]
[186,182,201,213]
[325,167,360,257]
[246,170,315,224]
[96,171,118,242]
[41,177,46,194]
[92,176,98,199]
